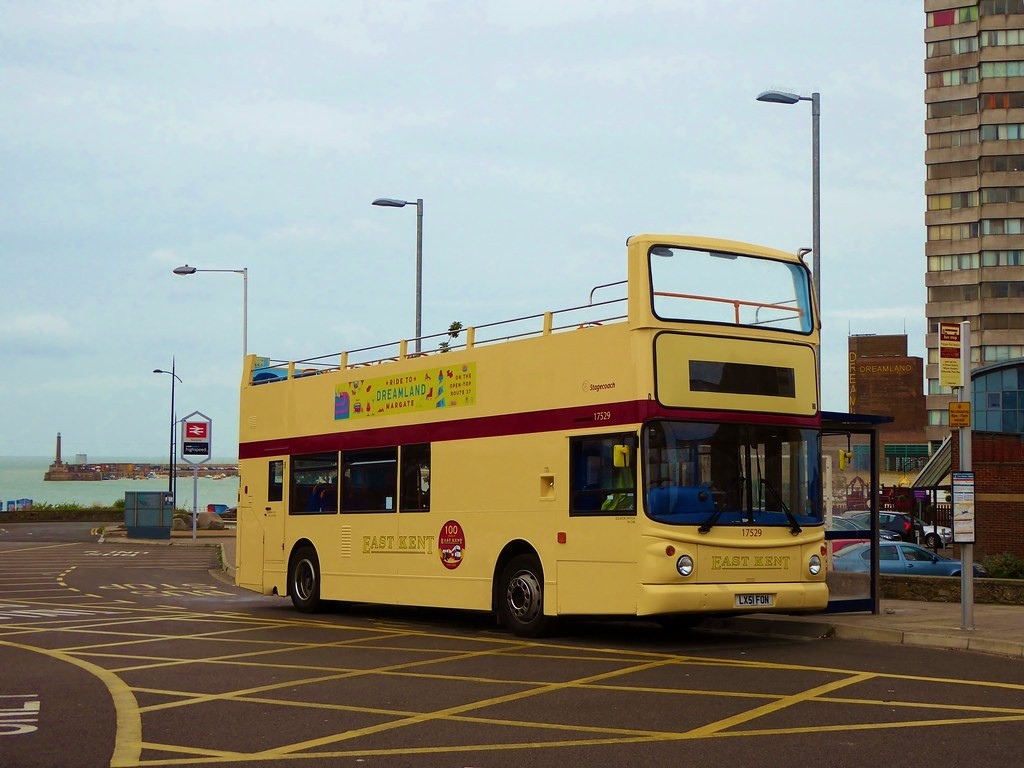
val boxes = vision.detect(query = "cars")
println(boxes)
[832,541,989,579]
[915,519,952,548]
[823,515,902,542]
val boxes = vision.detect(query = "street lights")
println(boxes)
[154,356,184,510]
[755,87,823,406]
[174,266,248,352]
[371,198,423,357]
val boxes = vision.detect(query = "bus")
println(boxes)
[236,234,830,636]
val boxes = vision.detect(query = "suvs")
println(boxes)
[841,510,924,542]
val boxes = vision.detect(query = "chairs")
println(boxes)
[350,362,372,369]
[407,353,429,359]
[378,358,398,364]
[318,488,338,512]
[578,321,603,330]
[302,369,323,377]
[308,483,333,512]
